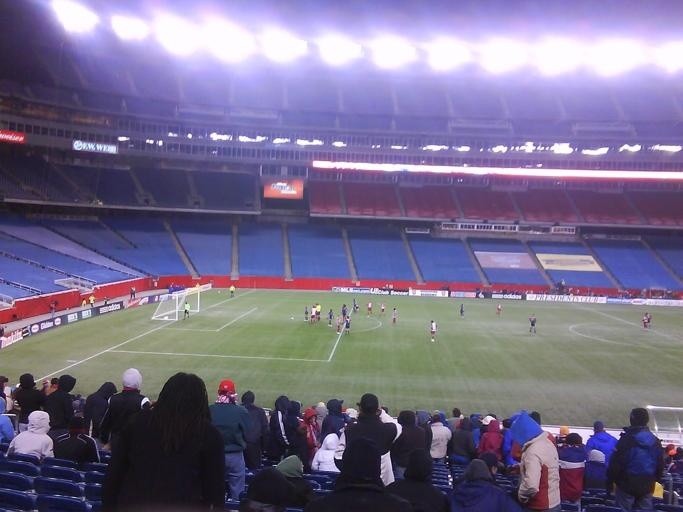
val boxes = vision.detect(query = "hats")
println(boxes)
[219,380,234,394]
[360,393,378,412]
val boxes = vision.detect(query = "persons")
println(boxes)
[3,362,683,512]
[101,372,225,512]
[50,281,235,321]
[306,278,682,343]
